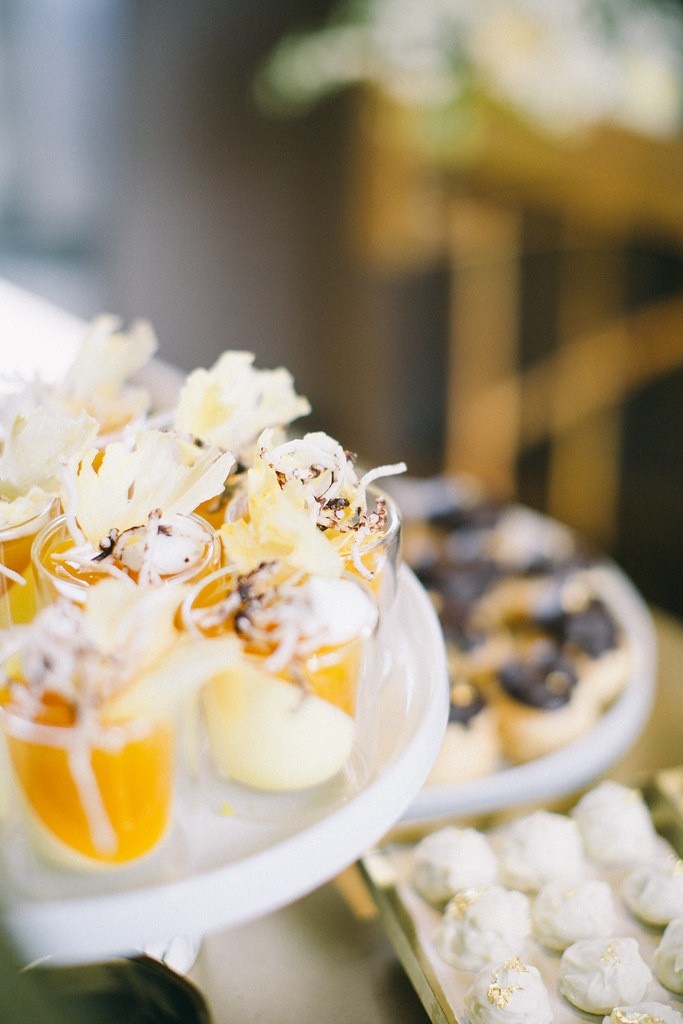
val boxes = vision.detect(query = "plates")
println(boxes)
[375,476,659,818]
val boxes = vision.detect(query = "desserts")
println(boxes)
[0,322,683,1024]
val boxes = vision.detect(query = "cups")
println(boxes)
[0,328,404,865]
